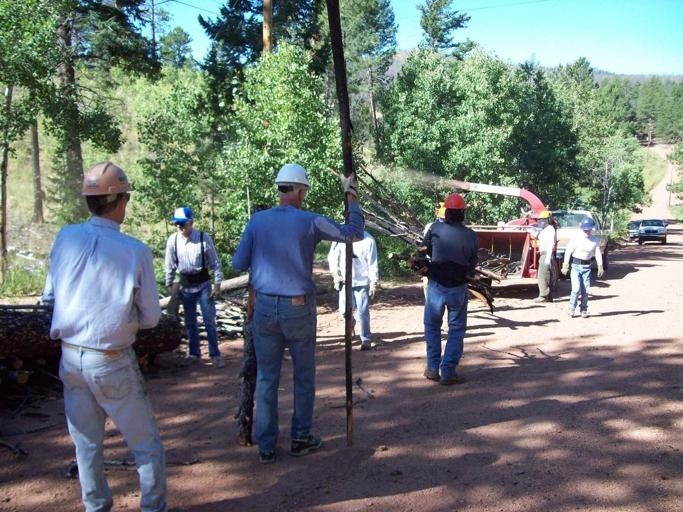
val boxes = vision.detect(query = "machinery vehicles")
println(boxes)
[464,188,559,294]
[406,179,559,292]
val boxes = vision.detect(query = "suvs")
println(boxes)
[637,218,668,245]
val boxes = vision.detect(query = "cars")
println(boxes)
[626,219,641,241]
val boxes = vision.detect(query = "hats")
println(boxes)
[170,208,192,224]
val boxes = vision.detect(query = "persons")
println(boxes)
[328,215,382,351]
[41,162,167,512]
[560,216,605,318]
[232,163,365,465]
[423,192,478,385]
[422,207,448,301]
[530,210,557,302]
[163,206,227,368]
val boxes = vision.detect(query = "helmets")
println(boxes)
[444,194,465,210]
[81,162,133,195]
[580,219,595,232]
[537,212,549,218]
[276,164,310,187]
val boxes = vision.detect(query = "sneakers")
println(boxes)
[568,308,575,317]
[440,375,465,385]
[425,369,440,379]
[581,311,587,318]
[360,341,372,350]
[180,354,199,366]
[261,448,277,463]
[534,295,553,302]
[212,357,226,369]
[290,439,322,457]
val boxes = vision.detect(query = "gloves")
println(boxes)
[340,171,357,199]
[368,282,376,300]
[561,263,569,276]
[597,266,604,277]
[333,281,341,291]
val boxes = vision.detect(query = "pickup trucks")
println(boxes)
[548,208,607,279]
